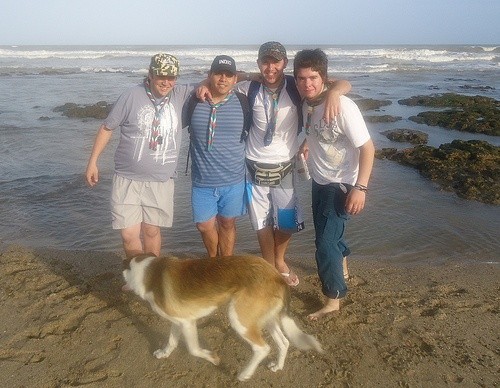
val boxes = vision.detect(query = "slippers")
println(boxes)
[280,269,299,287]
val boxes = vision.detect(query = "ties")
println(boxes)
[144,77,172,150]
[305,82,328,136]
[260,73,286,146]
[206,92,232,150]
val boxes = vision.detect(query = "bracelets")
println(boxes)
[352,183,369,193]
[244,71,251,81]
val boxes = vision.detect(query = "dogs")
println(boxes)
[114,249,324,382]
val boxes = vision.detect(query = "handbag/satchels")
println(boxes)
[244,155,299,189]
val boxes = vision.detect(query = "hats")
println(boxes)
[258,41,287,62]
[209,54,237,76]
[149,54,180,77]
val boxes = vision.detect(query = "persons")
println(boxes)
[86,39,265,292]
[195,41,353,287]
[181,54,252,257]
[293,47,375,320]
